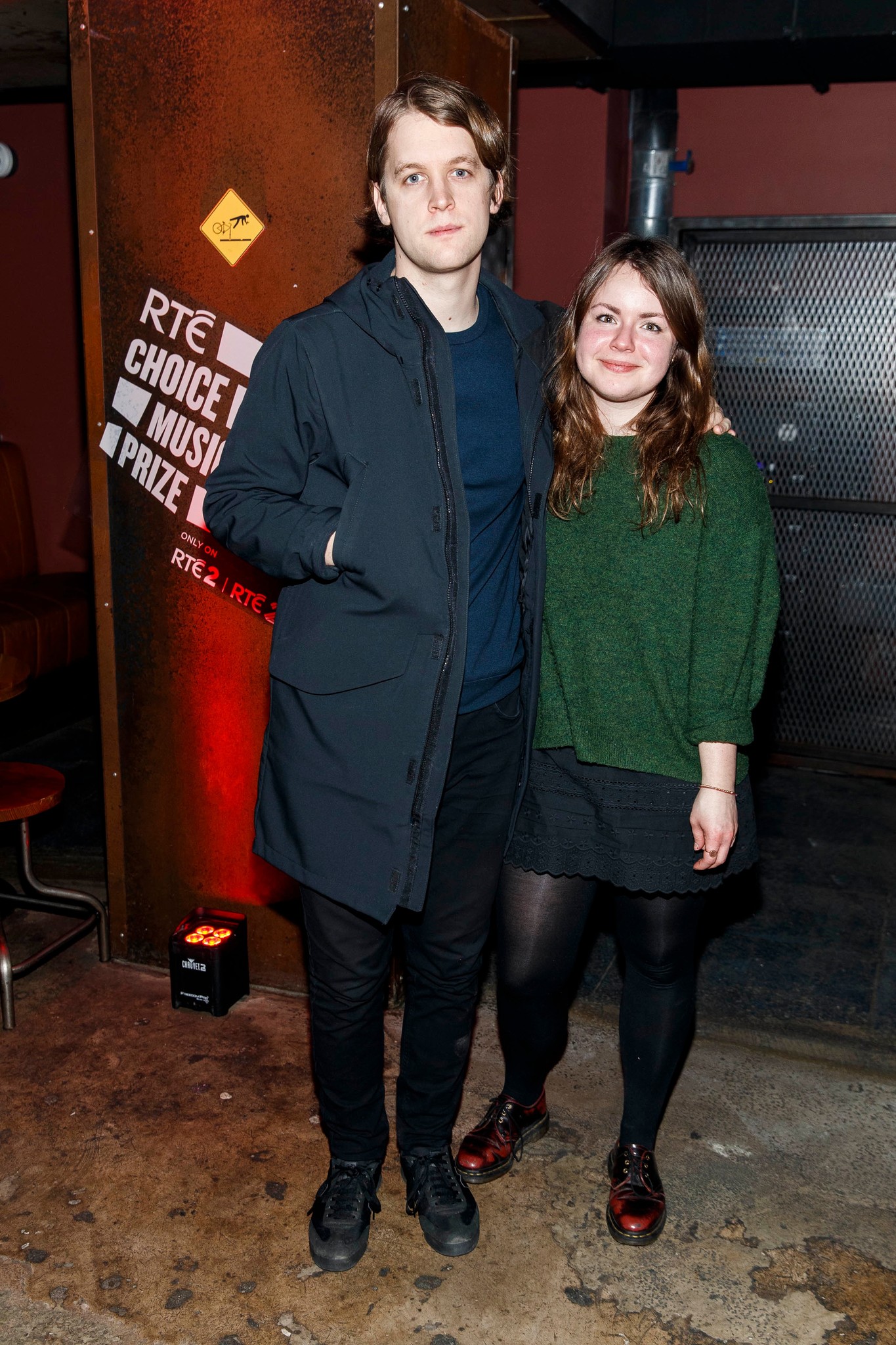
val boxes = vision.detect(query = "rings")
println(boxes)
[705,845,721,857]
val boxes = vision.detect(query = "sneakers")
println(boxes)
[606,1137,668,1247]
[455,1088,550,1185]
[307,1157,380,1273]
[400,1147,477,1256]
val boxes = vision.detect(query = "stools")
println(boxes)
[0,761,108,1030]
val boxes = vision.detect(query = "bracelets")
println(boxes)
[700,784,738,794]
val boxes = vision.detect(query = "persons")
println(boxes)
[455,232,784,1246]
[204,74,737,1273]
